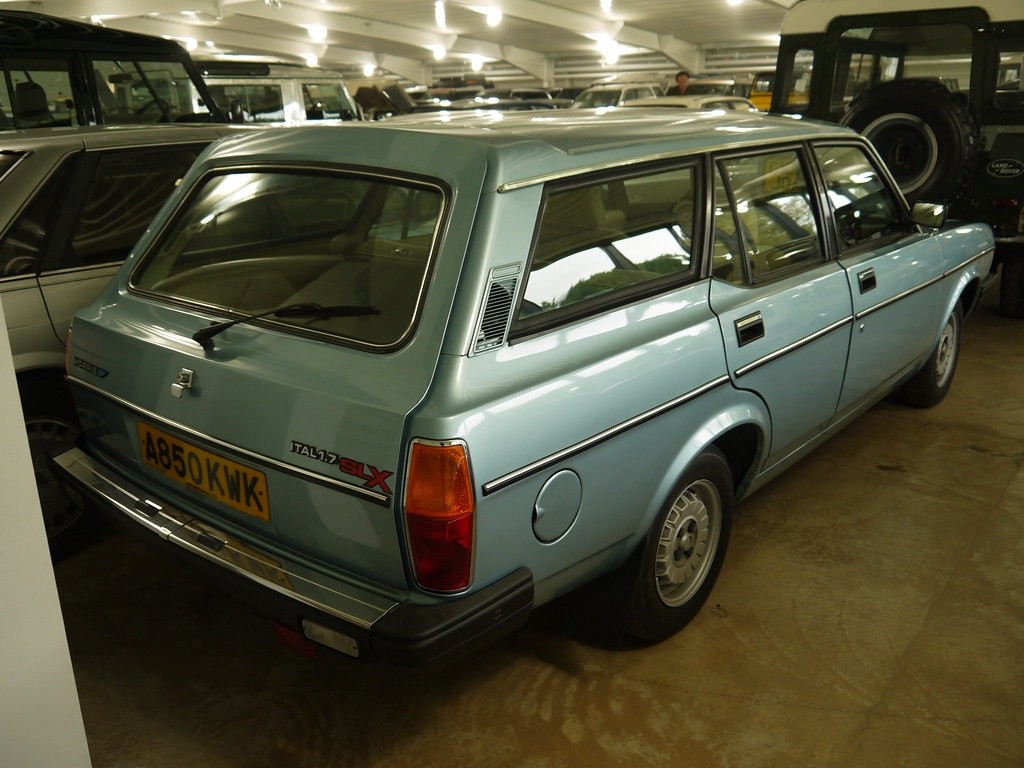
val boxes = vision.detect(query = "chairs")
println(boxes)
[534,185,645,272]
[675,187,791,286]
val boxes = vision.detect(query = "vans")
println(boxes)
[0,9,244,136]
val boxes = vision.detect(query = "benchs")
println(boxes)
[352,237,665,304]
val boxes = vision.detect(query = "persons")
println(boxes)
[665,71,697,96]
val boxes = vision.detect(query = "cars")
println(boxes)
[188,46,812,132]
[51,109,997,680]
[0,123,274,373]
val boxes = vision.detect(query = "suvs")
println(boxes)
[768,0,1023,319]
